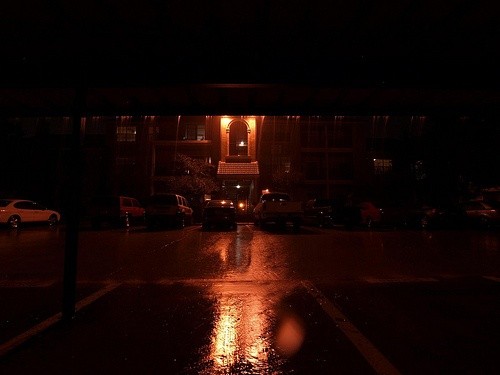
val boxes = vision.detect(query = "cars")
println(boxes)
[0,198,61,229]
[201,200,238,230]
[359,196,437,232]
[464,185,500,226]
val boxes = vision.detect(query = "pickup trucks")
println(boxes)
[253,188,305,229]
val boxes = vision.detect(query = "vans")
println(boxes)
[88,194,194,227]
